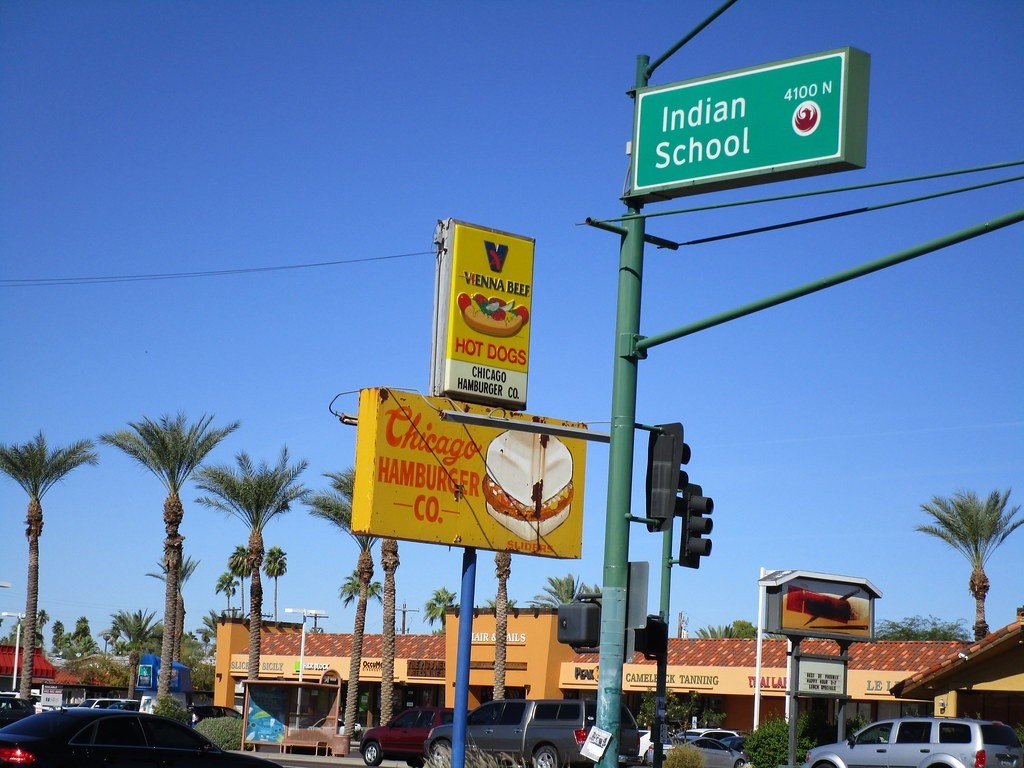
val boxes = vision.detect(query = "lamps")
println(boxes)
[939,699,944,709]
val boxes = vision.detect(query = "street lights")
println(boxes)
[284,607,329,730]
[1,611,40,692]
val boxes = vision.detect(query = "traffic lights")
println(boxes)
[646,422,692,532]
[678,483,714,570]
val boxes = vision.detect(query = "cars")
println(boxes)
[0,692,284,768]
[637,728,753,768]
[359,706,471,767]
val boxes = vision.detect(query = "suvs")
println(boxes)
[423,698,640,768]
[801,714,1024,768]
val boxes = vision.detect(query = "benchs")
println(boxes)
[279,740,328,757]
